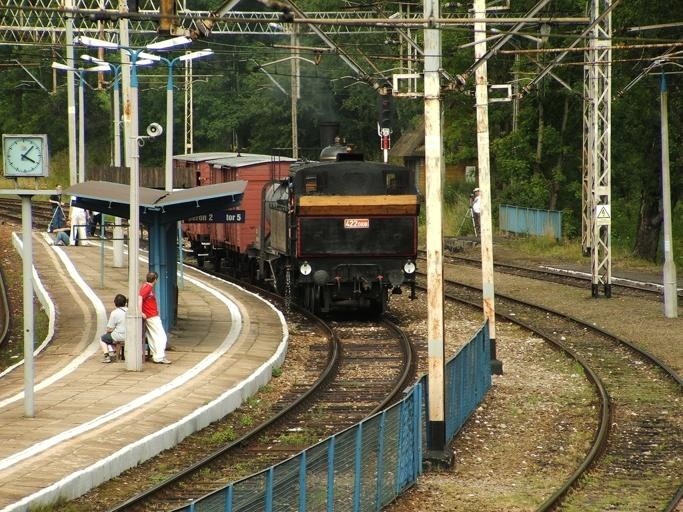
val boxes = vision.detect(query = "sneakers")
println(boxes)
[161,359,172,364]
[100,357,111,363]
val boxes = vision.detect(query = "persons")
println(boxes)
[47,182,65,229]
[90,210,106,237]
[136,272,173,365]
[471,188,481,235]
[97,293,128,363]
[47,219,78,246]
[84,209,93,238]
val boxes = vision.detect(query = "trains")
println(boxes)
[175,152,420,322]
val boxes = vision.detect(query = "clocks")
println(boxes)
[2,133,49,177]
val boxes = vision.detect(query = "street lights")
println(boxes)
[53,54,155,265]
[78,36,192,372]
[124,47,213,191]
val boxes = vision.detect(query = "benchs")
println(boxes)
[116,338,153,363]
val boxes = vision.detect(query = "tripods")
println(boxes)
[455,195,478,237]
